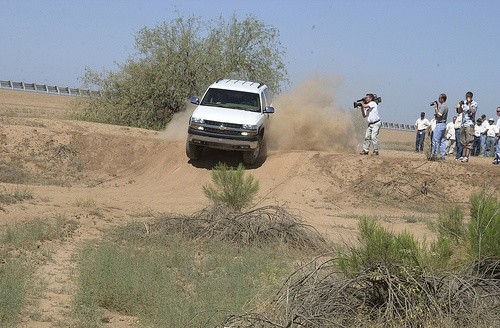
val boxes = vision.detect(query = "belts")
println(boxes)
[438,122,445,123]
[369,119,380,124]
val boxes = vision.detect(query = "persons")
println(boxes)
[357,94,383,155]
[414,91,500,165]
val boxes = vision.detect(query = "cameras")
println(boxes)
[430,101,437,106]
[461,101,464,104]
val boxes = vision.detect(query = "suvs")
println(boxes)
[185,78,275,167]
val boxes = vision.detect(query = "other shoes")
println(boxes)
[360,150,369,155]
[455,157,464,160]
[373,150,379,155]
[461,157,469,162]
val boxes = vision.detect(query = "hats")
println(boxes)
[421,112,425,115]
[488,118,494,121]
